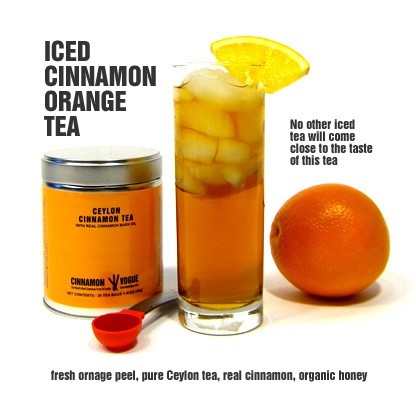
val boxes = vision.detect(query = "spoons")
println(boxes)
[91,290,178,353]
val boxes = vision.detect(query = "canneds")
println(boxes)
[41,149,162,317]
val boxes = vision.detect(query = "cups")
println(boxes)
[173,64,266,336]
